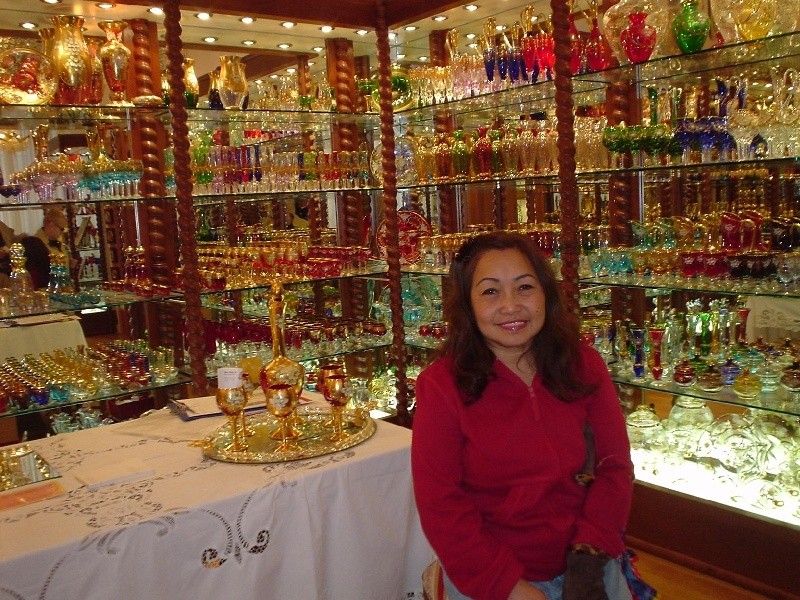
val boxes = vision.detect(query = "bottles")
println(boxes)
[396,116,606,185]
[38,15,130,106]
[184,55,332,114]
[260,279,304,438]
[579,297,800,516]
[588,1,800,71]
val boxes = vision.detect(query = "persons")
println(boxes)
[0,208,68,289]
[411,232,636,600]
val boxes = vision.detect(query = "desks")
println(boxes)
[0,383,440,600]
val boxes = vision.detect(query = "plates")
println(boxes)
[375,213,431,264]
[369,137,419,185]
[380,279,435,329]
[0,48,58,105]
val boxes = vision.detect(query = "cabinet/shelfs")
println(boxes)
[0,0,800,600]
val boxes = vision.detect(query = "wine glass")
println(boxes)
[165,145,369,195]
[197,242,311,289]
[357,33,554,114]
[216,387,248,452]
[602,109,759,170]
[417,221,611,272]
[325,374,353,440]
[265,384,303,453]
[0,160,143,204]
[588,246,800,296]
[317,366,347,427]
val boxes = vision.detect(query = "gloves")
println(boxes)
[563,553,610,600]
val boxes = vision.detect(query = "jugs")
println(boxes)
[634,211,800,251]
